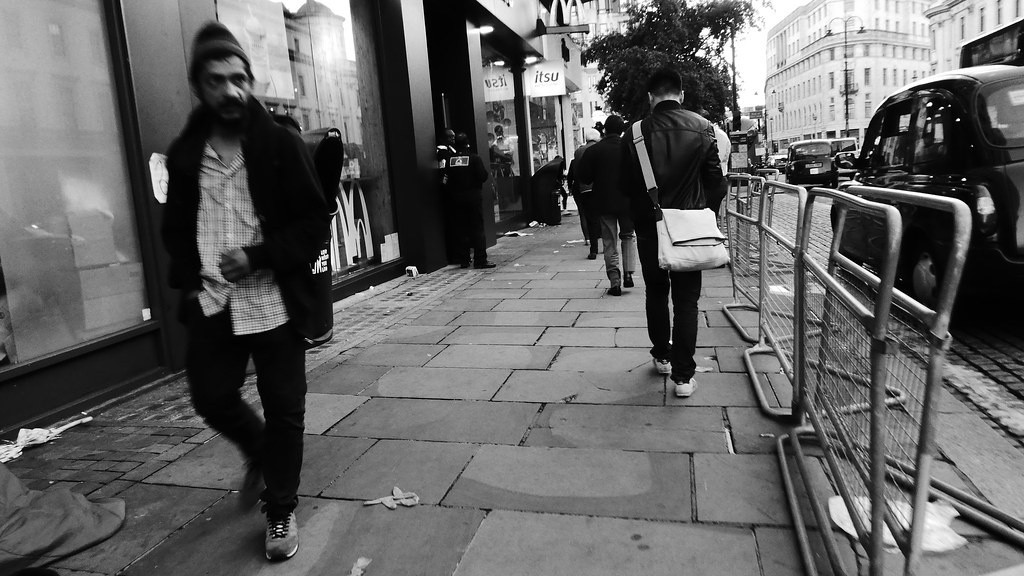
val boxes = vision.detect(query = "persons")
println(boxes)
[574,129,602,259]
[162,22,327,562]
[579,114,636,297]
[566,158,591,245]
[619,65,728,397]
[487,132,510,170]
[435,128,496,268]
[492,124,515,155]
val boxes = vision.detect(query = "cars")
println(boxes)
[829,137,859,181]
[830,64,1024,338]
[781,139,839,189]
[764,155,788,175]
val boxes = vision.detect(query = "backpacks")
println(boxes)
[299,127,343,201]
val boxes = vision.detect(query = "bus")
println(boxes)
[955,15,1024,69]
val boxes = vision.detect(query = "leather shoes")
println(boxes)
[624,272,634,288]
[608,287,621,296]
[474,259,497,268]
[461,258,473,268]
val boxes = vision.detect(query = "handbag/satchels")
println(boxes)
[655,208,733,271]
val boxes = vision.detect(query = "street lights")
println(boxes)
[826,16,867,139]
[755,83,777,161]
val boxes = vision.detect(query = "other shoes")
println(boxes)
[588,254,597,259]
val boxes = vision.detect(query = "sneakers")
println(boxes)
[654,357,672,374]
[674,378,698,397]
[265,507,299,561]
[240,464,266,505]
